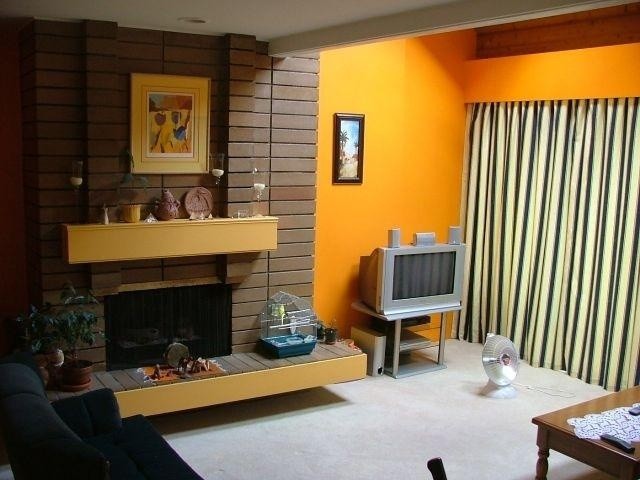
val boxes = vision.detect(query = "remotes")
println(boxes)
[601,434,635,453]
[629,405,640,415]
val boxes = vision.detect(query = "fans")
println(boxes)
[478,332,522,401]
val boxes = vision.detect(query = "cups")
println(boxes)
[237,209,250,219]
[448,226,462,246]
[388,228,400,248]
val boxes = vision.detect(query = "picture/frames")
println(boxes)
[128,71,211,176]
[334,113,363,184]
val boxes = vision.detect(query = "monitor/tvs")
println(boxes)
[359,243,466,316]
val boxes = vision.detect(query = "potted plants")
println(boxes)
[14,282,110,392]
[118,147,147,222]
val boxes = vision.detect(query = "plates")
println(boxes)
[185,186,214,218]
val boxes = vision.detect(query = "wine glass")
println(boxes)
[253,168,266,218]
[70,160,85,225]
[207,153,224,220]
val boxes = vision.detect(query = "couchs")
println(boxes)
[0,363,205,480]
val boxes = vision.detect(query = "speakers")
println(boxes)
[448,226,461,244]
[388,229,400,247]
[414,232,435,246]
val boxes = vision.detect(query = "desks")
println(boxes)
[531,385,640,480]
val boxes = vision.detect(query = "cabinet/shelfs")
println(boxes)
[351,308,462,378]
